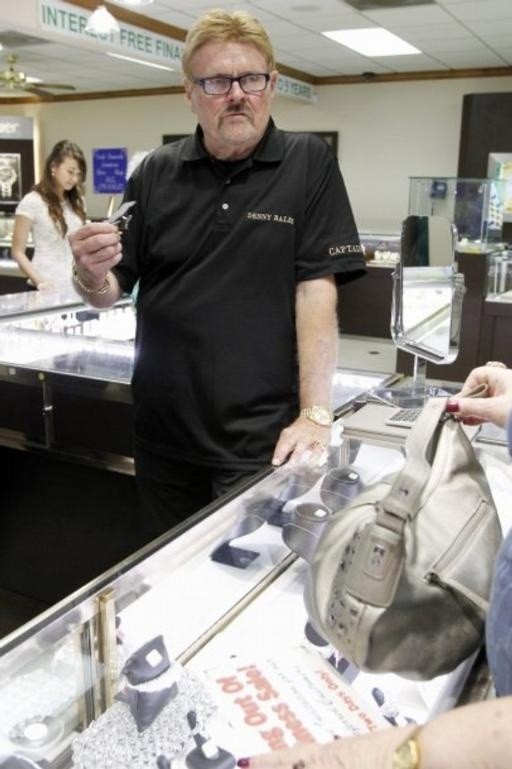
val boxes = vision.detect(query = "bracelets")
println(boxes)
[71,262,110,296]
[125,660,183,692]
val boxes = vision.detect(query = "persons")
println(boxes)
[241,362,510,768]
[10,141,86,291]
[69,7,368,538]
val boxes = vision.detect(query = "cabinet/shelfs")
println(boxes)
[1,284,141,343]
[327,231,403,340]
[347,366,509,458]
[1,323,395,607]
[409,169,508,383]
[0,424,492,769]
[477,286,511,372]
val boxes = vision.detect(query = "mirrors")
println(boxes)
[387,214,466,365]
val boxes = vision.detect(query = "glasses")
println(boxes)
[192,73,269,95]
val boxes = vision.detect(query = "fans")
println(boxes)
[1,72,74,105]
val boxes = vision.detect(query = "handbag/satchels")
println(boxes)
[305,396,502,682]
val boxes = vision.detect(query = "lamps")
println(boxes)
[84,0,122,40]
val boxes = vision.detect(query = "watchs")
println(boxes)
[392,722,424,768]
[300,404,333,427]
